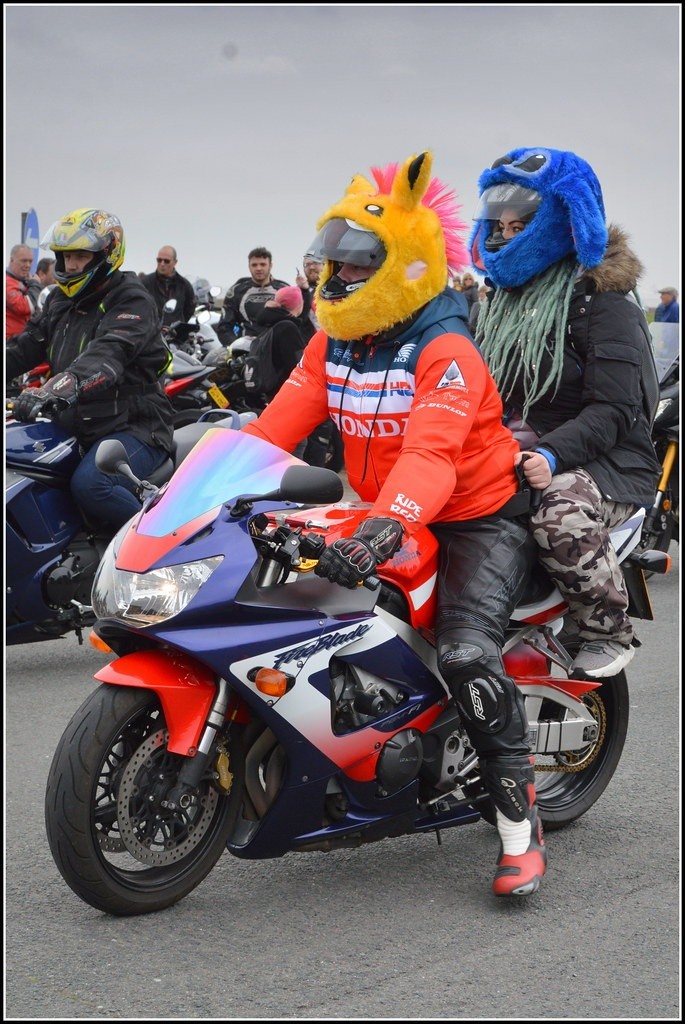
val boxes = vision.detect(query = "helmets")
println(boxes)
[50,209,125,301]
[485,177,542,254]
[315,216,388,300]
[232,337,256,374]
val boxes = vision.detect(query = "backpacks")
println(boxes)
[244,320,306,395]
[565,293,660,430]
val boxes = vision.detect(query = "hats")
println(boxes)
[276,286,302,311]
[659,286,678,296]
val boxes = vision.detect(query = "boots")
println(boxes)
[478,755,546,896]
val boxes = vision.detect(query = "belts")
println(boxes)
[90,382,161,398]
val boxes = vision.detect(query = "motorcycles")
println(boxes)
[5,286,256,430]
[43,427,670,915]
[5,386,258,646]
[643,322,680,582]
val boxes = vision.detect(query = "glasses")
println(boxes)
[157,258,171,264]
[303,262,319,267]
[251,263,267,268]
[464,278,472,280]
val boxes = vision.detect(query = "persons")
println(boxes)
[6,208,174,531]
[654,287,679,323]
[257,286,313,460]
[217,247,292,346]
[36,257,57,287]
[468,147,661,681]
[296,242,324,338]
[140,245,196,342]
[453,273,491,338]
[6,244,45,339]
[232,218,551,897]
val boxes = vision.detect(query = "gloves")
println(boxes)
[315,518,403,588]
[13,372,78,425]
[198,301,221,312]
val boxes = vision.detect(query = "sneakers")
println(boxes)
[568,639,634,678]
